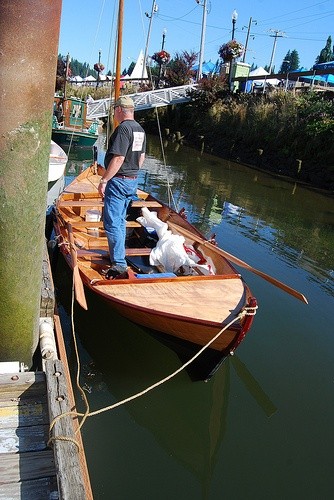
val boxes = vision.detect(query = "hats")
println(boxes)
[113,96,135,109]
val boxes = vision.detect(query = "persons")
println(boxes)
[99,96,147,276]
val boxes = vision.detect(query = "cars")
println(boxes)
[189,78,198,84]
[158,81,169,89]
[253,82,269,95]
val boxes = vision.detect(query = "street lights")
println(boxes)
[96,48,102,92]
[229,9,238,86]
[157,27,167,85]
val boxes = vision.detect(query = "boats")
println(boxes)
[52,55,99,151]
[47,139,69,218]
[54,164,258,356]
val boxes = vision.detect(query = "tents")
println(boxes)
[192,60,221,73]
[71,75,96,85]
[295,74,334,88]
[249,67,279,86]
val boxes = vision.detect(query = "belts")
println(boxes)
[115,173,138,179]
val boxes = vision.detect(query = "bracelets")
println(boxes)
[100,178,106,183]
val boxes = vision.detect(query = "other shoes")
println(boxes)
[103,267,126,276]
